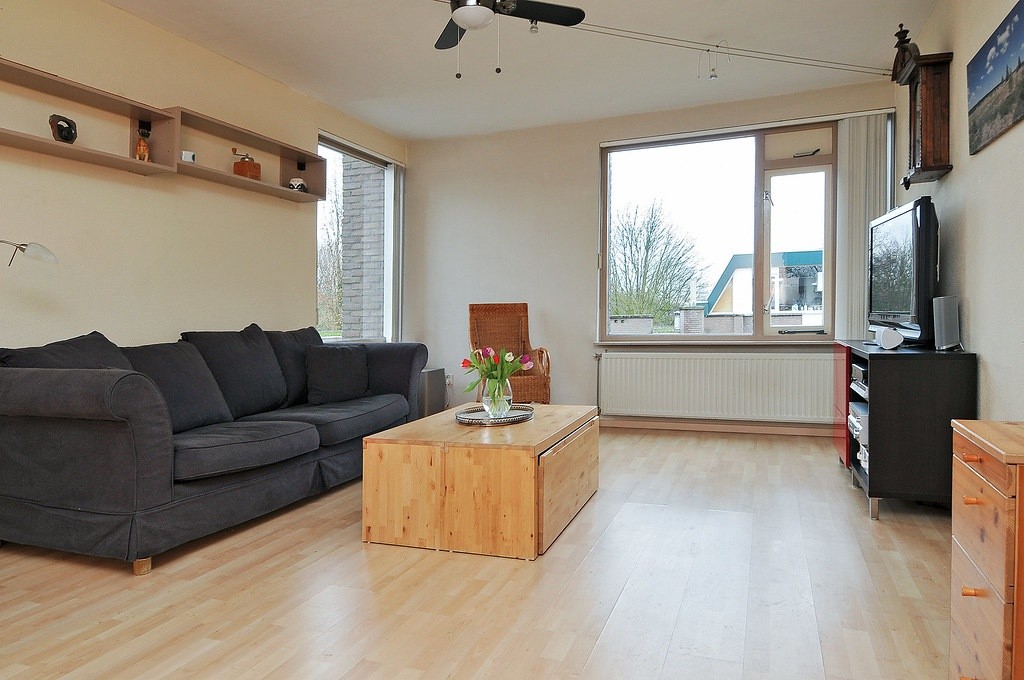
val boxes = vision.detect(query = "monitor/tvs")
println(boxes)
[867,196,939,347]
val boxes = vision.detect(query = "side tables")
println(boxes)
[419,367,446,418]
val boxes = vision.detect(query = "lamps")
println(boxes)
[449,0,496,29]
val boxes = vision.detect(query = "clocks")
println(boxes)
[901,50,955,188]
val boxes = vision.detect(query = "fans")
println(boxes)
[429,0,586,51]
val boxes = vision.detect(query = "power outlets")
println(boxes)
[444,373,453,385]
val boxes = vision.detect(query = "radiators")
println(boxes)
[593,351,836,425]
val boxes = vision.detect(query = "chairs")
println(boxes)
[468,300,551,404]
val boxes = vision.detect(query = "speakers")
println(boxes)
[932,296,965,353]
[875,328,904,349]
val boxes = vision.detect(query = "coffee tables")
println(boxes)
[361,401,601,564]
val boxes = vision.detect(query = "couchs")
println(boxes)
[0,341,427,576]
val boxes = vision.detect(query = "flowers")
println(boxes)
[458,344,534,411]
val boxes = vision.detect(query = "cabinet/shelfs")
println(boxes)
[0,58,177,184]
[847,341,978,522]
[947,419,1024,680]
[164,105,328,203]
[832,339,871,468]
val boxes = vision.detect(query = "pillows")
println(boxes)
[0,330,134,372]
[263,325,324,410]
[117,340,233,433]
[305,342,374,407]
[180,322,288,418]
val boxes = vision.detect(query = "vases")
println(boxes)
[479,379,514,417]
